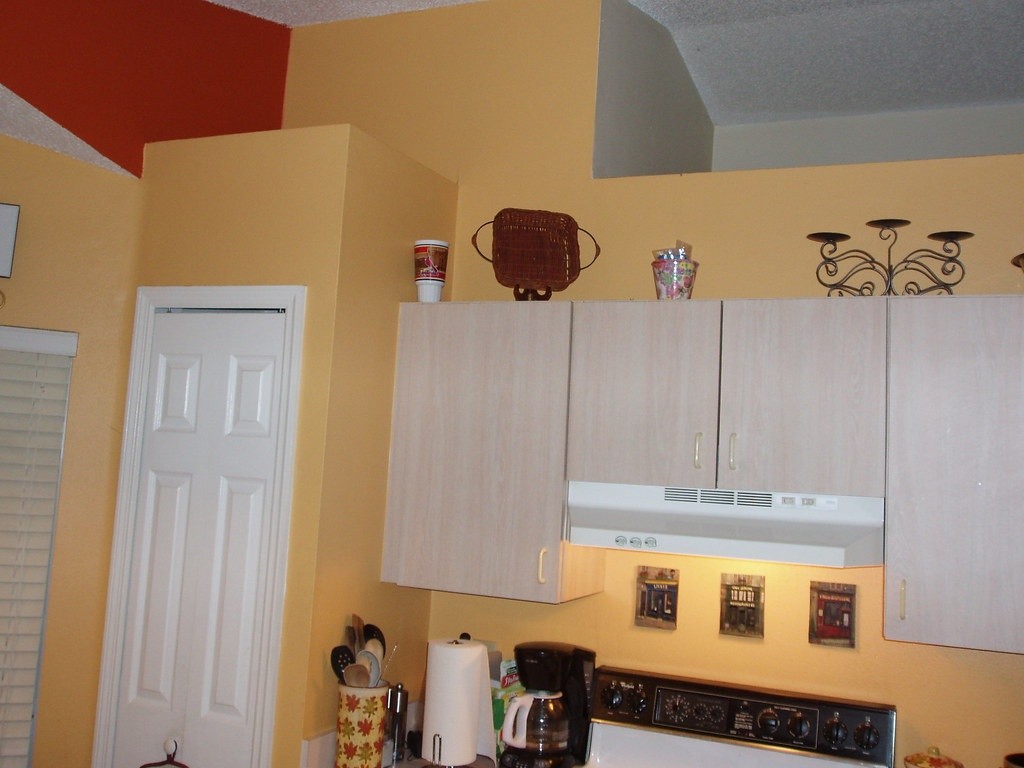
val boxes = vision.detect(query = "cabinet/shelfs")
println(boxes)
[379,301,605,607]
[566,298,887,499]
[881,295,1024,655]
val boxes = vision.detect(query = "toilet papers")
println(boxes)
[421,639,497,768]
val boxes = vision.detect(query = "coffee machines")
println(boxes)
[498,640,597,768]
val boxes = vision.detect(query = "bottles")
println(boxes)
[387,683,408,761]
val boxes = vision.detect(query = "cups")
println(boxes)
[414,239,449,302]
[650,260,699,299]
[905,746,965,768]
[334,679,389,768]
[1003,752,1024,768]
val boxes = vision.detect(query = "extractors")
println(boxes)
[561,480,885,569]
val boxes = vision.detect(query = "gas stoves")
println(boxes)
[581,667,898,768]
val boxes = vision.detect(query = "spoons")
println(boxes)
[365,639,383,663]
[356,650,381,687]
[344,663,371,687]
